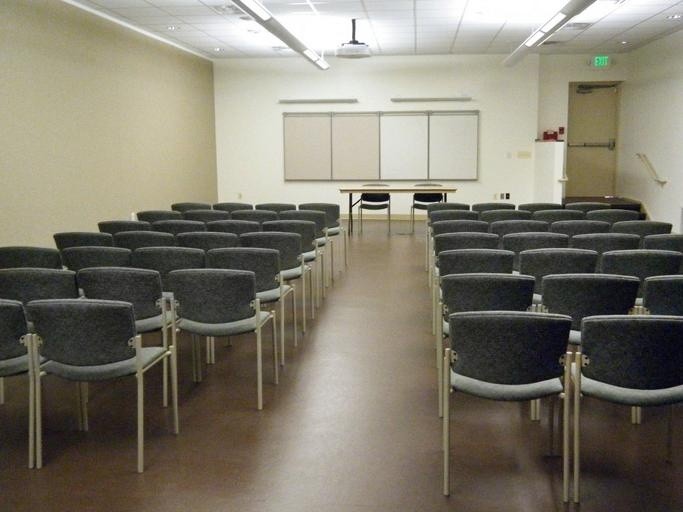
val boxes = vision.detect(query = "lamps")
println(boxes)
[498,0,598,70]
[232,0,332,73]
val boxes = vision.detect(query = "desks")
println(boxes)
[338,185,456,232]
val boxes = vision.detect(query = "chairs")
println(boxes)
[358,183,390,230]
[428,199,683,427]
[2,198,350,406]
[2,299,86,468]
[27,298,175,472]
[436,311,572,502]
[411,183,448,230]
[571,315,683,503]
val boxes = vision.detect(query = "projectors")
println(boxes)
[335,44,371,58]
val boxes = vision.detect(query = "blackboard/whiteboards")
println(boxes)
[283,111,478,182]
